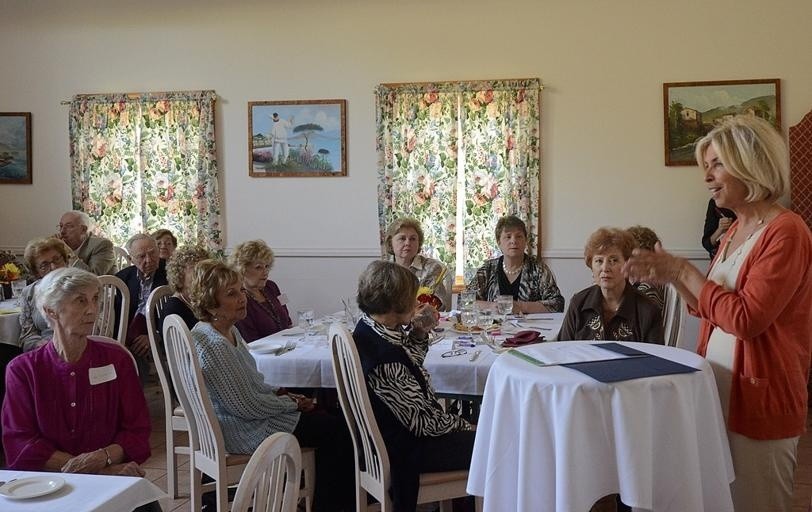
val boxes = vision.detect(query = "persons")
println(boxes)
[619,113,812,512]
[353,258,479,512]
[384,217,453,317]
[185,258,344,512]
[227,239,292,342]
[458,214,563,314]
[57,209,119,278]
[151,229,180,265]
[157,244,209,402]
[702,191,737,261]
[556,227,665,346]
[266,110,295,170]
[20,238,67,357]
[0,267,162,512]
[622,225,664,313]
[114,233,169,383]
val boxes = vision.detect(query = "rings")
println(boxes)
[143,346,148,349]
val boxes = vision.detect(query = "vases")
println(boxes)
[2,284,12,299]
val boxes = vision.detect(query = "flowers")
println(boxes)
[416,266,456,311]
[0,262,21,283]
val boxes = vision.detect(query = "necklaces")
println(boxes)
[501,259,524,275]
[601,293,624,312]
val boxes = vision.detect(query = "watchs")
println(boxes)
[102,445,114,469]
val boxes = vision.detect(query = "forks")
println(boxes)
[509,321,552,330]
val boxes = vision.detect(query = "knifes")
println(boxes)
[507,317,554,320]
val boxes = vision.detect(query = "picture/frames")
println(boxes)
[247,99,347,178]
[663,79,781,167]
[0,111,32,184]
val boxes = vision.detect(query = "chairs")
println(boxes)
[230,432,302,512]
[85,335,139,378]
[662,284,681,346]
[328,322,484,512]
[145,286,189,500]
[162,314,315,512]
[91,275,130,346]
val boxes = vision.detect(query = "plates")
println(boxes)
[0,475,66,502]
[250,343,282,354]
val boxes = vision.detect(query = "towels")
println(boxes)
[502,331,543,347]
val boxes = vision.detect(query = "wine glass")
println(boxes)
[411,284,513,345]
[298,296,363,343]
[10,279,28,308]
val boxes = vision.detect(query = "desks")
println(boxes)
[465,340,736,512]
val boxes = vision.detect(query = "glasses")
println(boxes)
[442,349,467,358]
[55,223,74,231]
[39,254,63,271]
